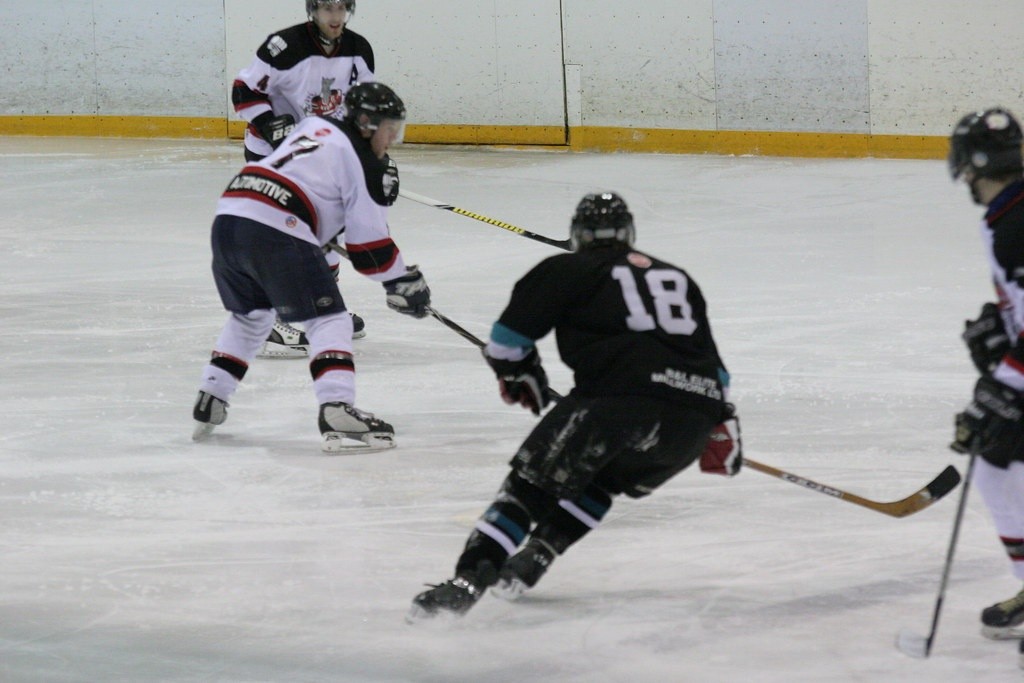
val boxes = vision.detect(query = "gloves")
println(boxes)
[381,159,400,206]
[482,344,551,417]
[960,302,1010,376]
[383,264,432,320]
[699,403,743,477]
[952,374,1024,468]
[261,114,296,151]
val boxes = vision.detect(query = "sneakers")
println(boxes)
[405,572,487,629]
[349,311,367,339]
[980,588,1024,641]
[489,541,555,602]
[317,401,397,452]
[190,389,230,440]
[254,314,308,358]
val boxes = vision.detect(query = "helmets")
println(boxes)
[947,108,1024,183]
[344,82,406,121]
[570,190,630,229]
[306,0,353,11]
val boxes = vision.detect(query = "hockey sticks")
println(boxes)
[896,432,980,661]
[397,189,578,252]
[327,239,490,350]
[503,378,961,519]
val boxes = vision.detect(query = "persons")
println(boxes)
[192,81,432,455]
[949,108,1024,668]
[406,192,743,628]
[232,0,375,358]
